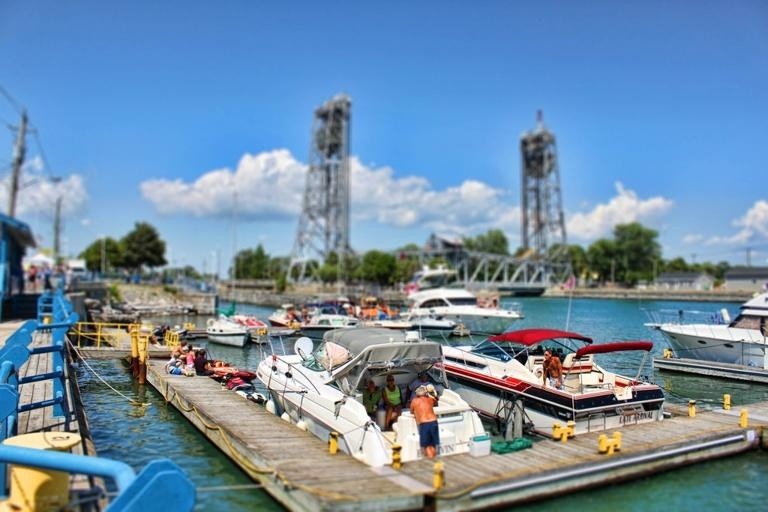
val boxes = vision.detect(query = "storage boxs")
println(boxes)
[468,432,492,457]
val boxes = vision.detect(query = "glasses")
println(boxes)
[545,354,549,356]
[388,379,394,382]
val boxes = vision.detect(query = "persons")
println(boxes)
[26,263,54,292]
[541,349,566,392]
[380,374,402,430]
[409,385,440,459]
[361,380,383,422]
[170,339,215,376]
[405,374,435,408]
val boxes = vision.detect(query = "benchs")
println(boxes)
[376,383,409,428]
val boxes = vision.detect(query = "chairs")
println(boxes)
[579,373,616,394]
